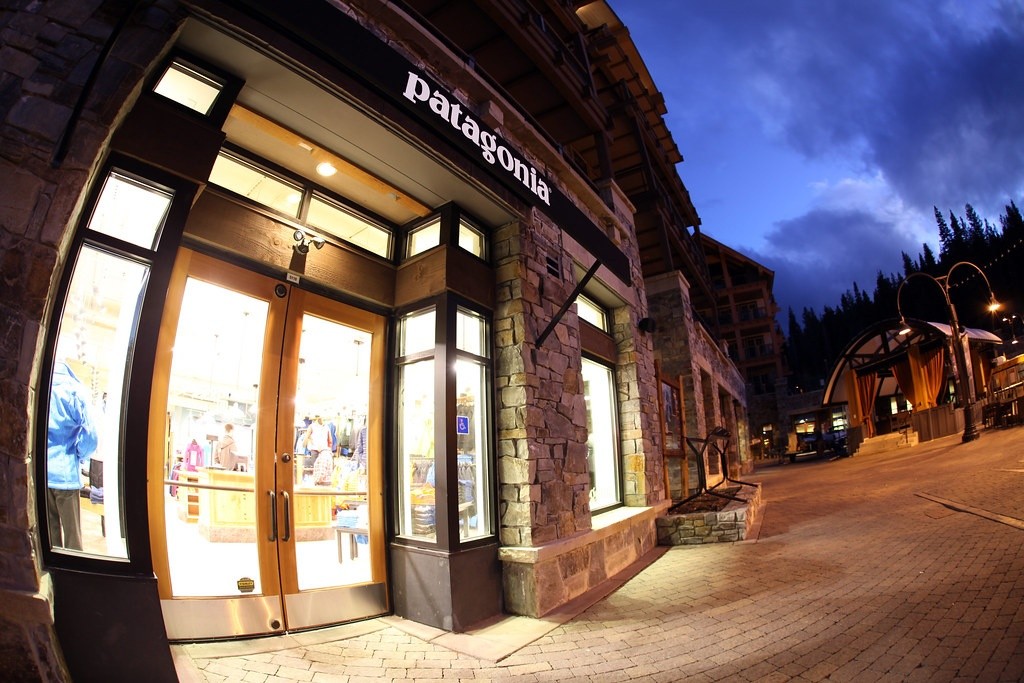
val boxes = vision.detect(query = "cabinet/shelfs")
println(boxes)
[196,469,335,543]
[174,470,200,522]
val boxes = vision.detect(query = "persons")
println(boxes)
[48,341,97,549]
[213,424,238,464]
[313,442,334,485]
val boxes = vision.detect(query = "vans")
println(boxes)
[987,352,1024,404]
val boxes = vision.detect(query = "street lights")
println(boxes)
[896,260,1003,444]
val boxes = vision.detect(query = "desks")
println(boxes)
[332,526,370,562]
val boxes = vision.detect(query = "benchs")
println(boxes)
[877,410,911,436]
